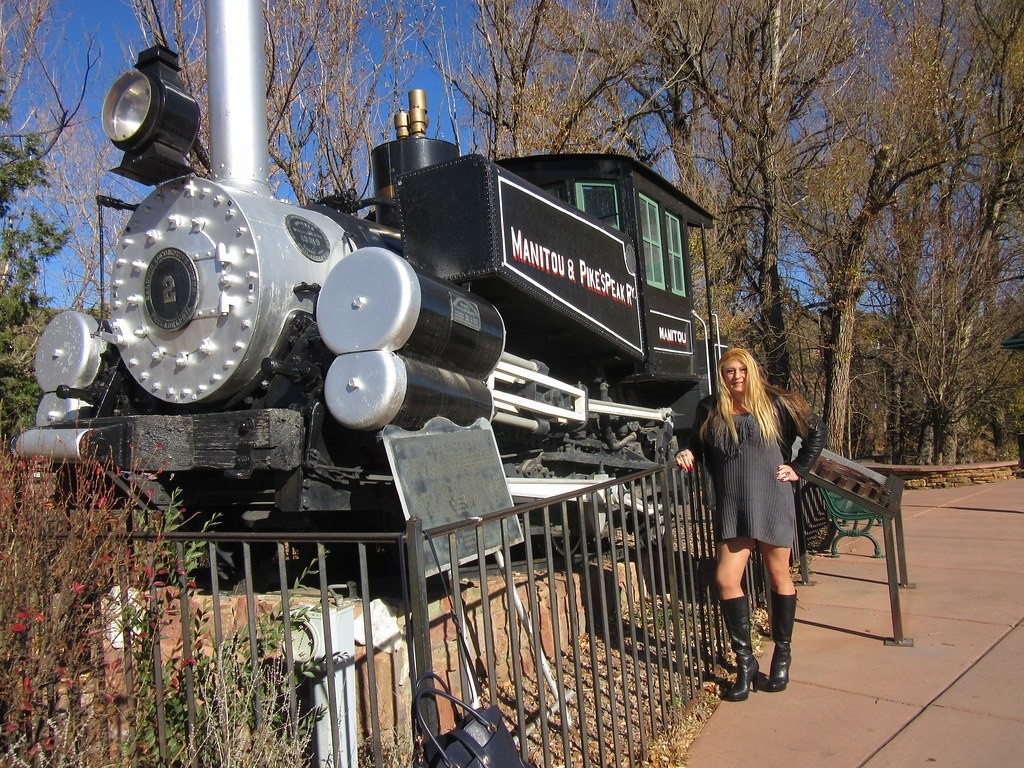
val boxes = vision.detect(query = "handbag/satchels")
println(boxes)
[412,672,534,768]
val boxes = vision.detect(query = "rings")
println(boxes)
[678,453,687,459]
[785,472,791,476]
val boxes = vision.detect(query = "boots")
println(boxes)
[721,594,759,701]
[767,589,797,692]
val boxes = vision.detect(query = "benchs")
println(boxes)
[816,487,885,560]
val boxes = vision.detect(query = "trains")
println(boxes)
[2,0,736,590]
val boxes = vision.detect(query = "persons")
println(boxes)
[674,349,829,702]
